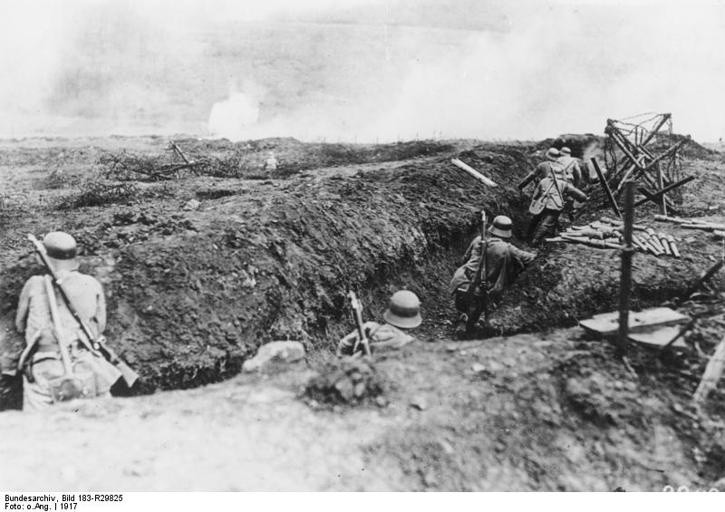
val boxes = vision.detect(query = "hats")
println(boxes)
[560,146,572,157]
[36,231,81,274]
[545,147,561,161]
[486,213,514,239]
[548,166,564,179]
[381,288,424,330]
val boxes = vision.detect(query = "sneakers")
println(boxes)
[455,312,469,337]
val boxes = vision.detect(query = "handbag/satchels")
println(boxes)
[63,337,123,399]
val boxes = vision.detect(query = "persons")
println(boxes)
[340,288,423,357]
[519,147,591,242]
[16,231,121,409]
[450,216,536,333]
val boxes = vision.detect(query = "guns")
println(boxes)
[346,290,371,355]
[26,233,138,390]
[482,211,496,312]
[19,337,43,382]
